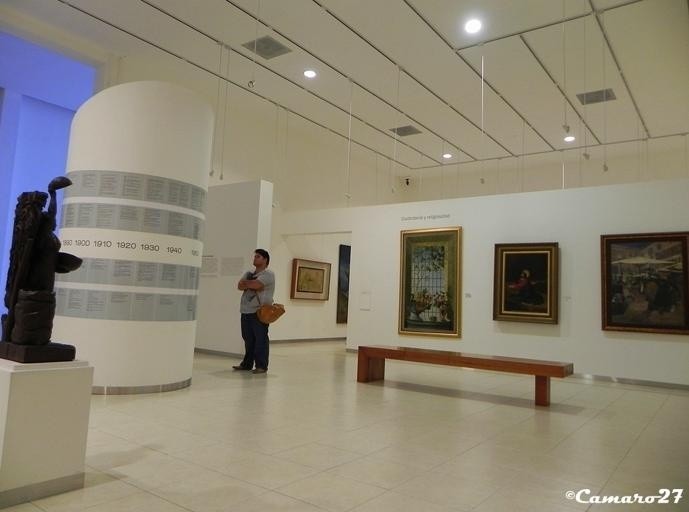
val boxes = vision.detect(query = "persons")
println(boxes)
[506,267,530,297]
[232,249,275,374]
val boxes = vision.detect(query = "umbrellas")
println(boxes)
[612,256,676,273]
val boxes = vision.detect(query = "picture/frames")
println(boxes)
[490,242,563,326]
[596,230,689,337]
[396,225,464,341]
[290,257,332,302]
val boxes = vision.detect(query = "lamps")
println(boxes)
[204,0,619,214]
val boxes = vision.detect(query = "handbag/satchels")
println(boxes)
[256,303,286,325]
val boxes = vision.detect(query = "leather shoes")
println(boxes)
[232,364,252,371]
[250,367,268,375]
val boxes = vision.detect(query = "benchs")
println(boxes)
[355,341,577,409]
